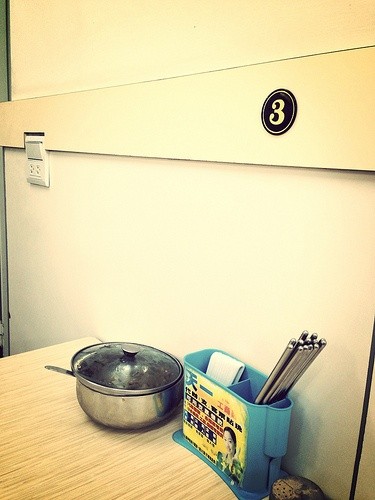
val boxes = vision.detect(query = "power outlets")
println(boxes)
[25,135,49,187]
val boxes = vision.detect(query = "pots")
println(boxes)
[70,340,184,431]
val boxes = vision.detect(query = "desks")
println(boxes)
[0,336,325,500]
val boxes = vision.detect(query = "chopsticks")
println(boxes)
[249,330,328,405]
